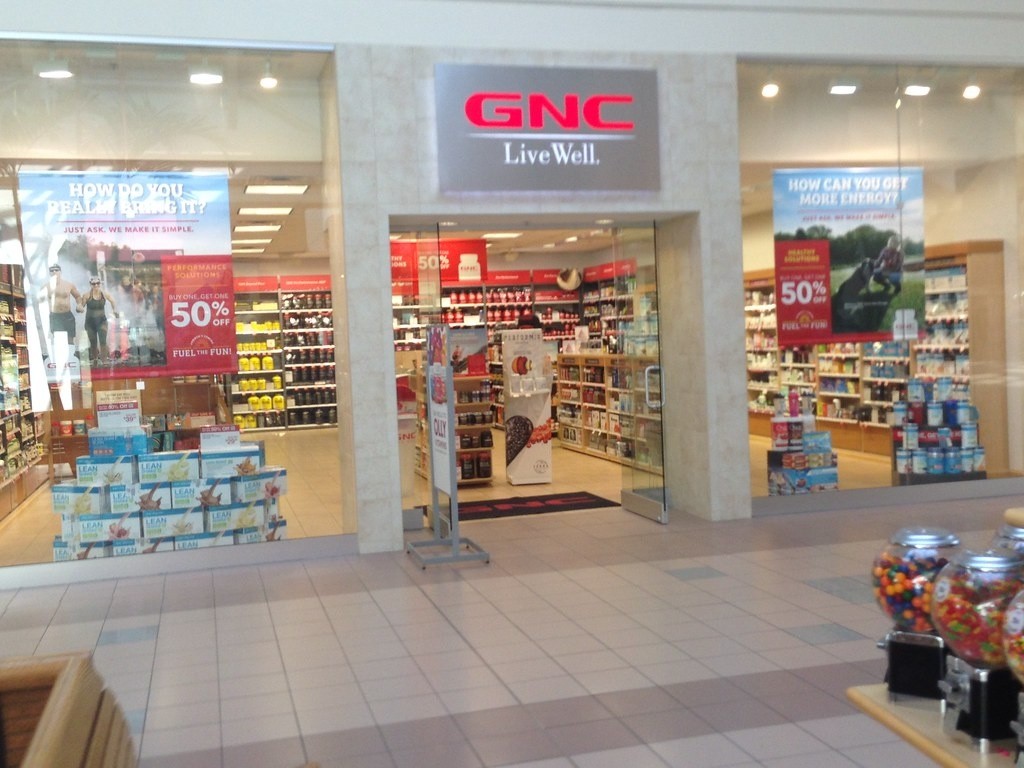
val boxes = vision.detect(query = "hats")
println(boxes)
[49,263,61,271]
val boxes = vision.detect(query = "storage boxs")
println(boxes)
[112,537,175,556]
[61,514,80,542]
[166,413,192,431]
[68,541,112,560]
[767,467,841,498]
[199,443,260,478]
[231,465,288,504]
[175,530,234,550]
[264,496,280,522]
[171,477,232,509]
[76,455,139,485]
[203,500,264,533]
[138,449,199,483]
[110,482,171,514]
[145,413,166,432]
[153,432,164,452]
[51,483,110,514]
[53,535,69,560]
[233,519,288,543]
[87,424,153,457]
[140,507,204,539]
[79,511,143,542]
[163,431,176,451]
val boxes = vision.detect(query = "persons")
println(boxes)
[75,275,119,368]
[871,234,904,294]
[29,263,84,367]
[113,274,167,365]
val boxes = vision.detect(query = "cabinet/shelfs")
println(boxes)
[483,270,533,364]
[0,263,50,521]
[279,275,338,431]
[228,276,287,434]
[598,256,639,338]
[532,269,580,339]
[581,265,602,335]
[637,239,1010,473]
[51,378,233,476]
[489,362,558,438]
[556,353,663,475]
[392,281,441,343]
[441,280,486,329]
[413,370,494,486]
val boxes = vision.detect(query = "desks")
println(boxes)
[847,682,1017,768]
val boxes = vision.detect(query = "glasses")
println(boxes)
[49,269,59,273]
[89,277,100,283]
[90,282,100,286]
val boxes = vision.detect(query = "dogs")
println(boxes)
[838,257,875,300]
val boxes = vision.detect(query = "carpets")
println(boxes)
[415,491,622,522]
